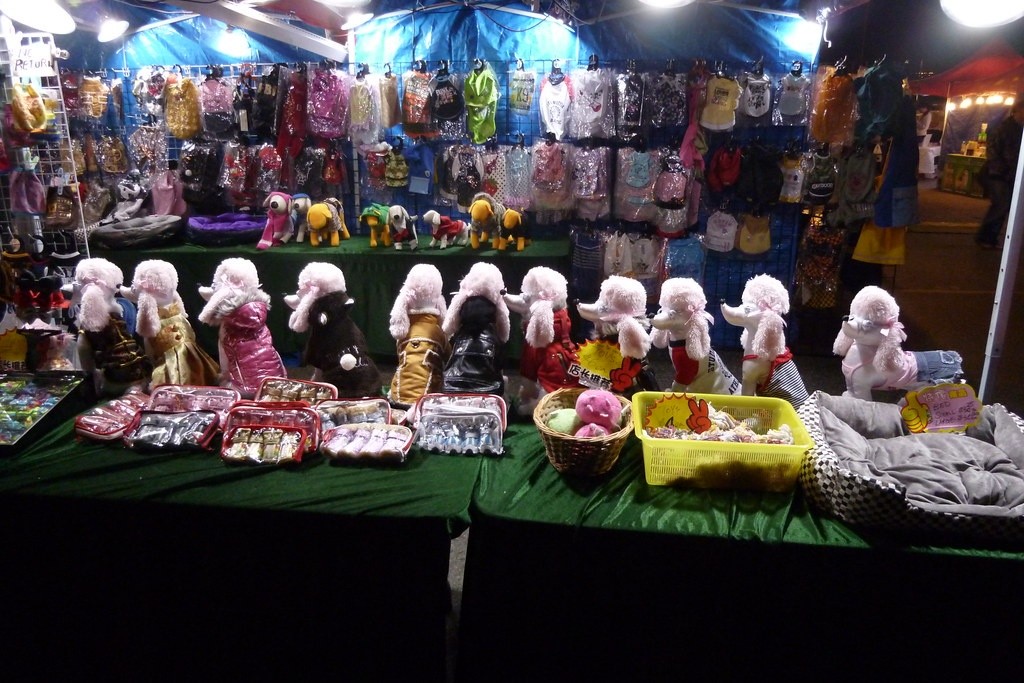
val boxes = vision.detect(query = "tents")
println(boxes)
[906,31,1024,141]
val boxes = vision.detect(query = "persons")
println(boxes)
[976,92,1023,249]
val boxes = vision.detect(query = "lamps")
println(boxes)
[92,7,131,43]
[940,0,1024,31]
[0,0,77,35]
[544,1,580,33]
[340,8,375,31]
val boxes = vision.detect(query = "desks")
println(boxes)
[473,406,1024,683]
[0,381,484,683]
[89,226,570,366]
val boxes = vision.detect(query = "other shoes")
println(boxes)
[974,235,1003,252]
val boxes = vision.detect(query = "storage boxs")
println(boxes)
[632,391,814,494]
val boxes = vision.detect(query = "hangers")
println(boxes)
[61,50,914,96]
[564,210,709,254]
[53,121,888,176]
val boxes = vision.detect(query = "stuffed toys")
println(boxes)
[256,192,295,252]
[468,192,514,249]
[115,260,222,391]
[283,262,385,401]
[499,208,531,250]
[575,388,624,431]
[500,267,582,415]
[719,273,811,410]
[831,285,963,402]
[440,262,512,409]
[574,423,611,437]
[387,265,454,409]
[422,211,468,249]
[386,205,418,250]
[648,278,741,396]
[196,256,289,400]
[359,202,393,249]
[291,194,313,243]
[544,409,582,436]
[60,257,151,396]
[573,275,660,394]
[307,197,350,246]
